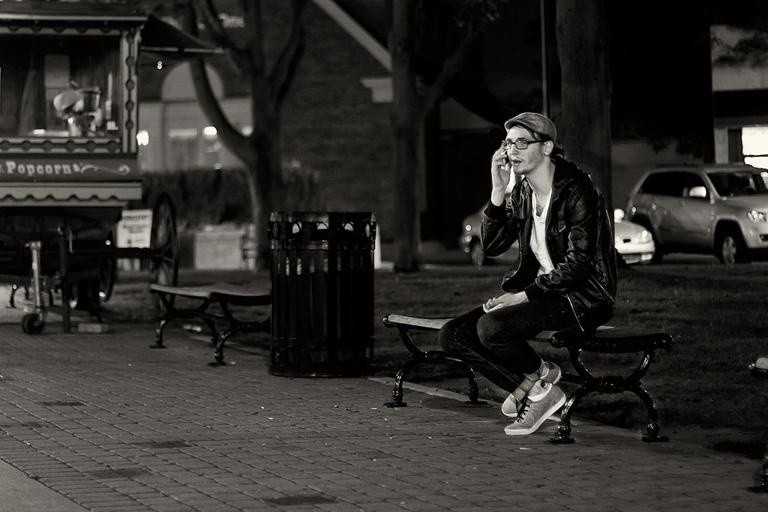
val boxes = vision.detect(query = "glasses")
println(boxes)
[506,138,546,150]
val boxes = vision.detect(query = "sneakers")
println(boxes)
[501,362,566,436]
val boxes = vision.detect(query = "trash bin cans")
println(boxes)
[264,209,377,378]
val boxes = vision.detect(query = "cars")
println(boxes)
[458,199,655,269]
[623,164,768,265]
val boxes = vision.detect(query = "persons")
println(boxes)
[438,112,616,434]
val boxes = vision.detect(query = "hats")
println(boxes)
[504,112,556,142]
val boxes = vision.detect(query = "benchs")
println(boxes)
[383,314,675,444]
[748,358,768,493]
[150,282,271,366]
